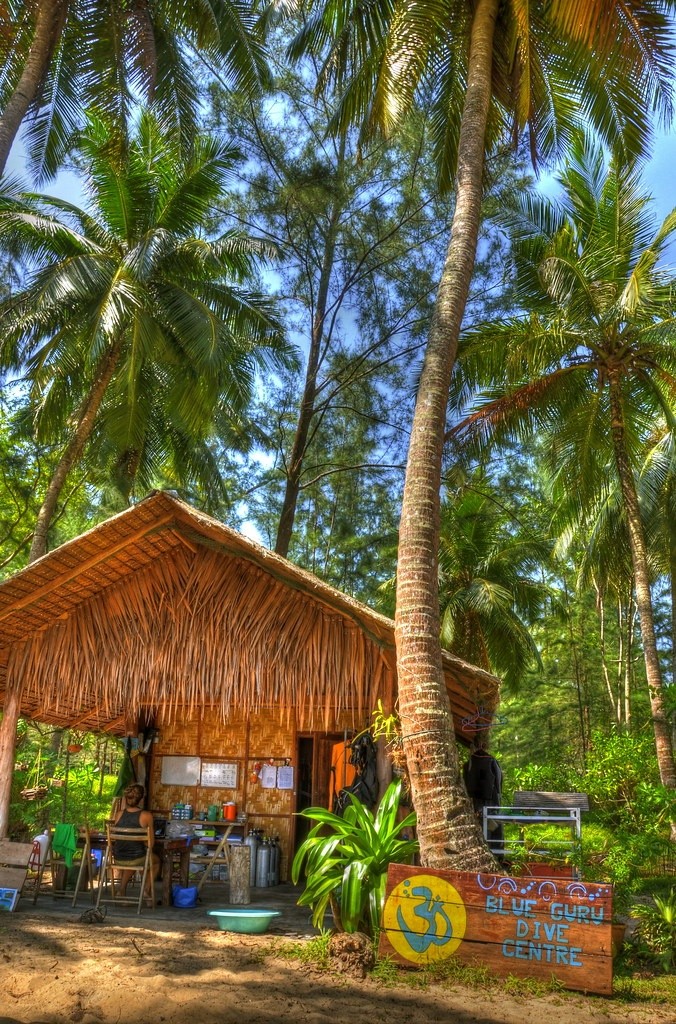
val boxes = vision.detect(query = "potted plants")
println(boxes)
[13,718,88,801]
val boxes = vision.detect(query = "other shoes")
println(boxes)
[116,891,129,907]
[143,891,153,907]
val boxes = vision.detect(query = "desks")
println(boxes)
[50,836,202,906]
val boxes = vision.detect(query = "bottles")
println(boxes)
[220,807,224,819]
[30,830,49,864]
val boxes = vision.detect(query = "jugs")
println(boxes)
[223,802,236,820]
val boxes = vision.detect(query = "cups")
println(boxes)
[199,813,205,820]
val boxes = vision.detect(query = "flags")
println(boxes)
[112,754,142,796]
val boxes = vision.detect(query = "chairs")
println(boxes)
[32,819,157,916]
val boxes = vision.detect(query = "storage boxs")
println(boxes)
[190,824,220,842]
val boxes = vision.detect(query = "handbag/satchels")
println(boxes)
[170,884,202,908]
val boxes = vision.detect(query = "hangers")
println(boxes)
[461,699,509,771]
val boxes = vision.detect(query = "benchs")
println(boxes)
[498,790,590,852]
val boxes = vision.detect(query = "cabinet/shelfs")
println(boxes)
[167,818,254,886]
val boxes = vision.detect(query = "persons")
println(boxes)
[112,785,159,906]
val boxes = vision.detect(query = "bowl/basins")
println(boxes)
[206,909,282,934]
